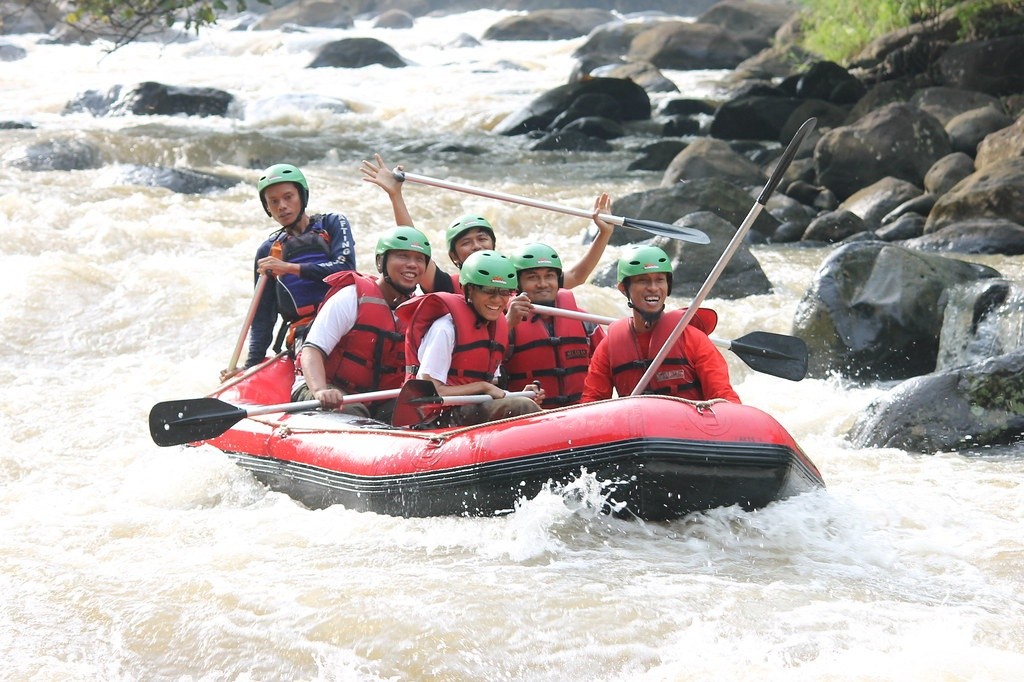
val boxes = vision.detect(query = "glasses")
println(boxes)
[468,283,514,296]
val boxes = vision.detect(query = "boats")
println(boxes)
[181,346,827,538]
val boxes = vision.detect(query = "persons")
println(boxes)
[293,153,741,427]
[220,163,356,383]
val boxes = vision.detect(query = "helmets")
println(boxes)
[445,216,496,268]
[512,243,564,288]
[616,246,673,297]
[459,250,518,289]
[374,225,431,274]
[257,163,310,217]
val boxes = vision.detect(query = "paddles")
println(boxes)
[390,171,713,245]
[147,388,402,448]
[631,115,820,397]
[392,376,542,428]
[226,265,274,379]
[524,303,811,384]
